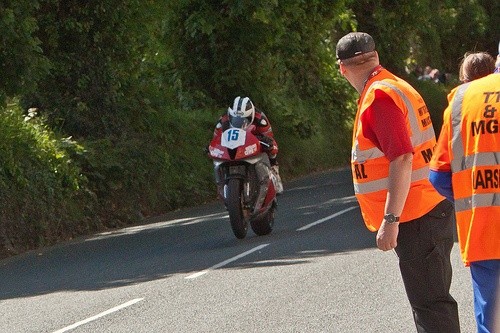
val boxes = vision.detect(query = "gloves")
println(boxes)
[258,135,273,151]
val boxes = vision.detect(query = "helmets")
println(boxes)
[227,96,255,131]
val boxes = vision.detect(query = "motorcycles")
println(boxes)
[207,115,284,240]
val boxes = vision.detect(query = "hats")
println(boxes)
[336,32,375,60]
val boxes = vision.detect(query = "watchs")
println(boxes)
[383,214,400,223]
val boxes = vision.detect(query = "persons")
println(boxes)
[415,67,453,86]
[428,43,500,333]
[459,52,496,83]
[212,96,278,207]
[335,32,461,333]
